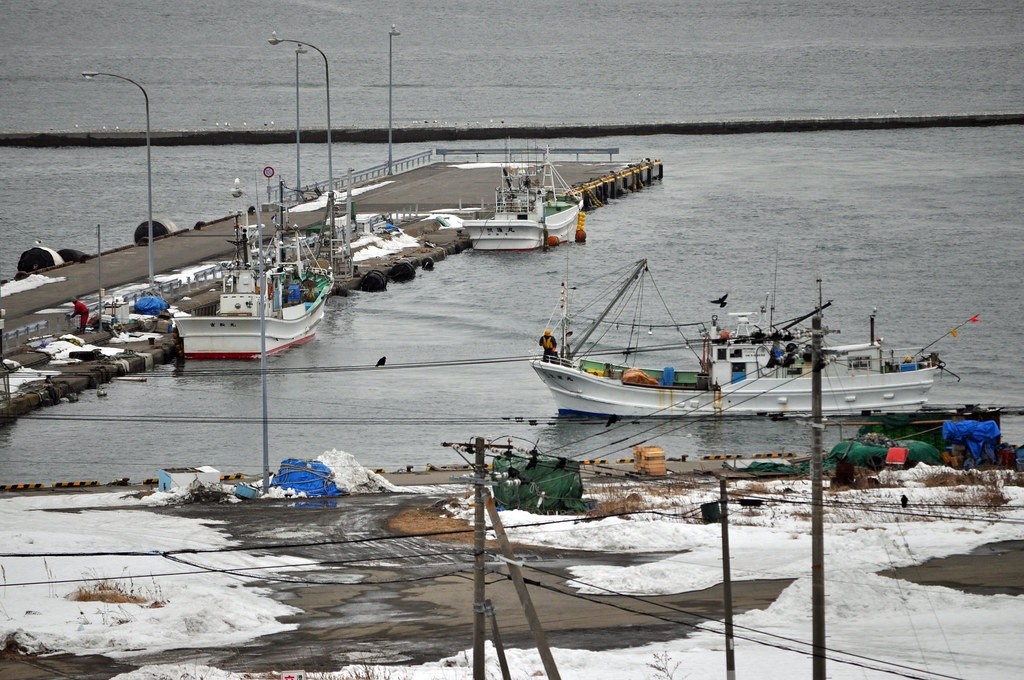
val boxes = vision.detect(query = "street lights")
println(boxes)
[294,42,309,201]
[386,21,401,177]
[267,37,337,242]
[81,69,154,290]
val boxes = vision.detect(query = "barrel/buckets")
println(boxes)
[941,444,965,468]
[148,338,155,345]
[700,502,720,524]
[664,366,674,386]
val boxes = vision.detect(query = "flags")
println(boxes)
[951,330,957,336]
[972,317,978,322]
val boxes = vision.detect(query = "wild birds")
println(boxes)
[900,494,908,508]
[376,356,387,367]
[710,293,729,308]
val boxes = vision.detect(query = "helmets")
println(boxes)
[545,329,551,336]
[71,297,77,301]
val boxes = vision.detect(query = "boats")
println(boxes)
[623,367,659,385]
[531,258,981,421]
[173,174,335,360]
[468,135,588,250]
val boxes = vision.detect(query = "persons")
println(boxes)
[539,329,557,362]
[70,296,89,331]
